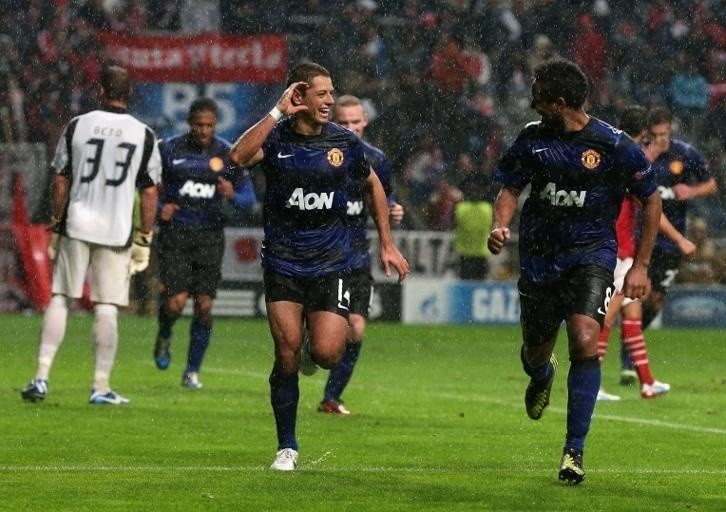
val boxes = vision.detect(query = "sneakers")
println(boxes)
[155,337,170,369]
[271,448,299,472]
[525,352,558,419]
[641,381,670,396]
[298,335,317,374]
[89,390,130,404]
[183,372,202,388]
[596,390,620,400]
[559,448,585,482]
[619,370,636,385]
[318,400,350,415]
[21,380,47,401]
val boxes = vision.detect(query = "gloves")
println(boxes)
[129,229,153,276]
[48,215,62,260]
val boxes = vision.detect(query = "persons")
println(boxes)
[152,97,255,390]
[595,104,671,401]
[486,59,662,482]
[314,95,405,415]
[620,104,717,384]
[20,65,161,405]
[1,2,724,313]
[229,61,409,472]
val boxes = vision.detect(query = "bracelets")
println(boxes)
[269,105,283,122]
